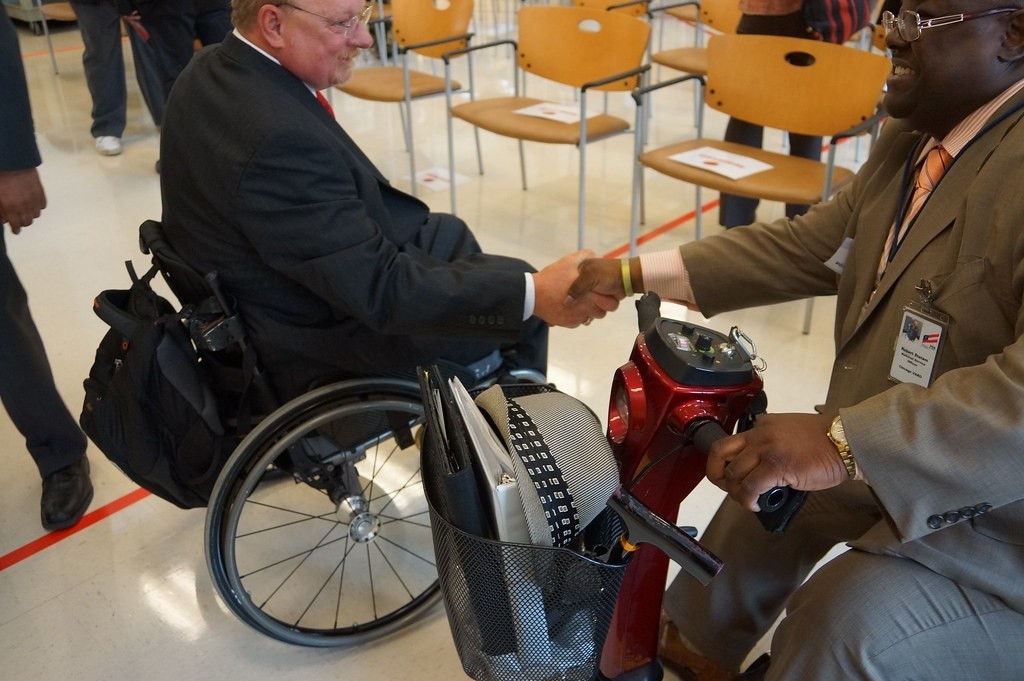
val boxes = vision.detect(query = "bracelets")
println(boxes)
[622,258,637,297]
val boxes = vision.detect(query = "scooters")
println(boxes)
[433,292,804,681]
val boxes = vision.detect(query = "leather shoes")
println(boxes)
[30,448,95,531]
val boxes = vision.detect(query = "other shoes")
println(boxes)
[93,135,125,157]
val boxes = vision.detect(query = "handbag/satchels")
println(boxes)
[79,277,227,511]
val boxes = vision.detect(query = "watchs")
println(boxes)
[827,412,855,481]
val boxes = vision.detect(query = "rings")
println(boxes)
[583,316,590,325]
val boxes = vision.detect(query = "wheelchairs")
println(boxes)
[91,218,550,652]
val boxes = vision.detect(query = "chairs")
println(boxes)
[659,0,744,128]
[36,0,78,74]
[443,6,652,250]
[630,34,893,335]
[328,0,484,198]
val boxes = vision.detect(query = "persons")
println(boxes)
[718,0,826,230]
[563,0,1024,681]
[0,0,94,535]
[69,0,233,158]
[158,1,618,403]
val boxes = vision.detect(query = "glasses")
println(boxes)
[276,1,374,38]
[878,8,1015,42]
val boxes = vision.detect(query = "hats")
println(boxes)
[477,388,621,588]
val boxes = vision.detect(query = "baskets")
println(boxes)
[421,384,630,681]
